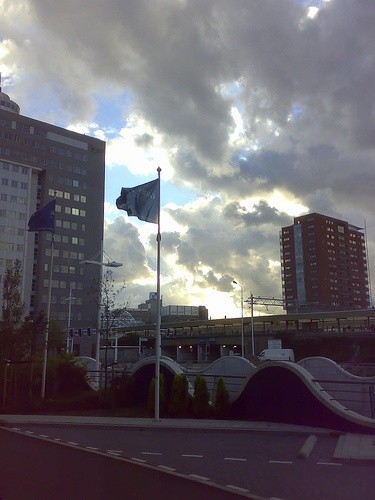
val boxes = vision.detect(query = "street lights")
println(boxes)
[79,239,123,390]
[233,281,244,359]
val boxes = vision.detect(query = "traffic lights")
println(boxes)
[87,328,90,336]
[78,329,81,337]
[70,329,73,337]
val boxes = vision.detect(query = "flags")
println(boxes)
[116,178,159,224]
[27,199,56,233]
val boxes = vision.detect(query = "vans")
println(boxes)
[258,349,294,362]
